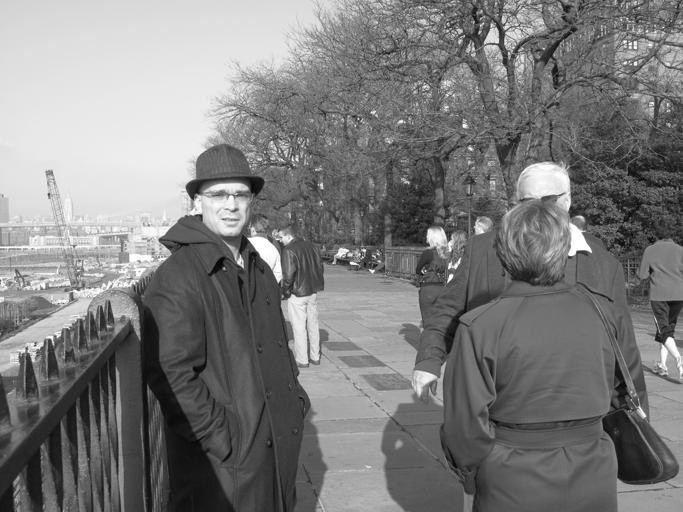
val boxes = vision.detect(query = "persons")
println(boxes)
[140,144,312,512]
[438,201,649,511]
[416,215,494,330]
[277,225,324,367]
[410,159,627,405]
[331,246,386,274]
[572,215,607,251]
[636,220,683,380]
[245,212,284,286]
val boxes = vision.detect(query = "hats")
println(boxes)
[183,143,265,199]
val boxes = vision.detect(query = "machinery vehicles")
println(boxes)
[12,267,31,291]
[46,170,84,291]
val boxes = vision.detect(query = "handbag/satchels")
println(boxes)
[599,399,681,488]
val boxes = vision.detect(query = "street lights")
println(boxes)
[463,172,476,240]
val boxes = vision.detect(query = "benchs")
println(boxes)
[321,250,378,272]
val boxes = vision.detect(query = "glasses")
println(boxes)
[197,189,254,200]
[518,192,568,206]
